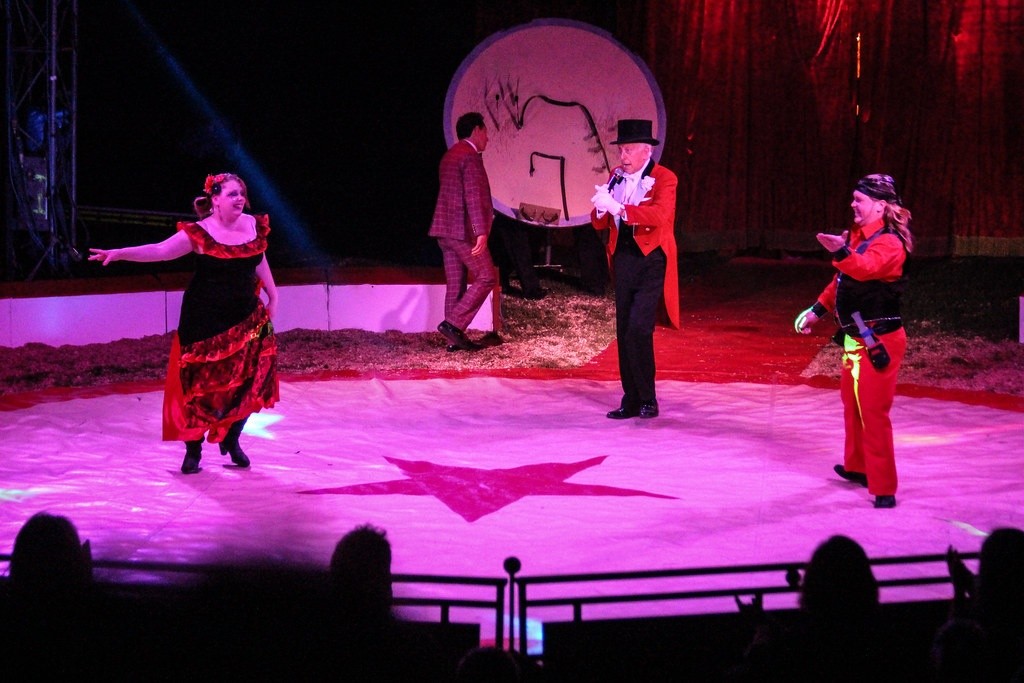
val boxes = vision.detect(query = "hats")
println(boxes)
[854,174,897,203]
[609,118,660,146]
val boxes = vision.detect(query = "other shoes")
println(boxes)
[437,320,474,349]
[588,285,606,296]
[447,343,460,351]
[523,289,547,298]
[834,464,868,487]
[503,285,513,293]
[875,495,896,508]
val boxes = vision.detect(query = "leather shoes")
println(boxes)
[640,400,658,418]
[606,399,640,418]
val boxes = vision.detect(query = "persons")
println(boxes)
[427,111,495,352]
[590,119,680,419]
[795,174,912,509]
[89,172,278,475]
[455,646,523,683]
[731,534,884,683]
[323,523,394,618]
[8,510,81,592]
[930,530,1024,683]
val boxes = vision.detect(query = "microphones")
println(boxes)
[607,168,623,192]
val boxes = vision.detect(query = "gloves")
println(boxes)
[596,184,609,211]
[590,184,621,216]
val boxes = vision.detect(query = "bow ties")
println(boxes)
[622,172,640,183]
[479,153,482,158]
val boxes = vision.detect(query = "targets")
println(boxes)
[442,18,667,228]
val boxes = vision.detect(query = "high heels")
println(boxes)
[219,428,250,467]
[181,437,205,473]
